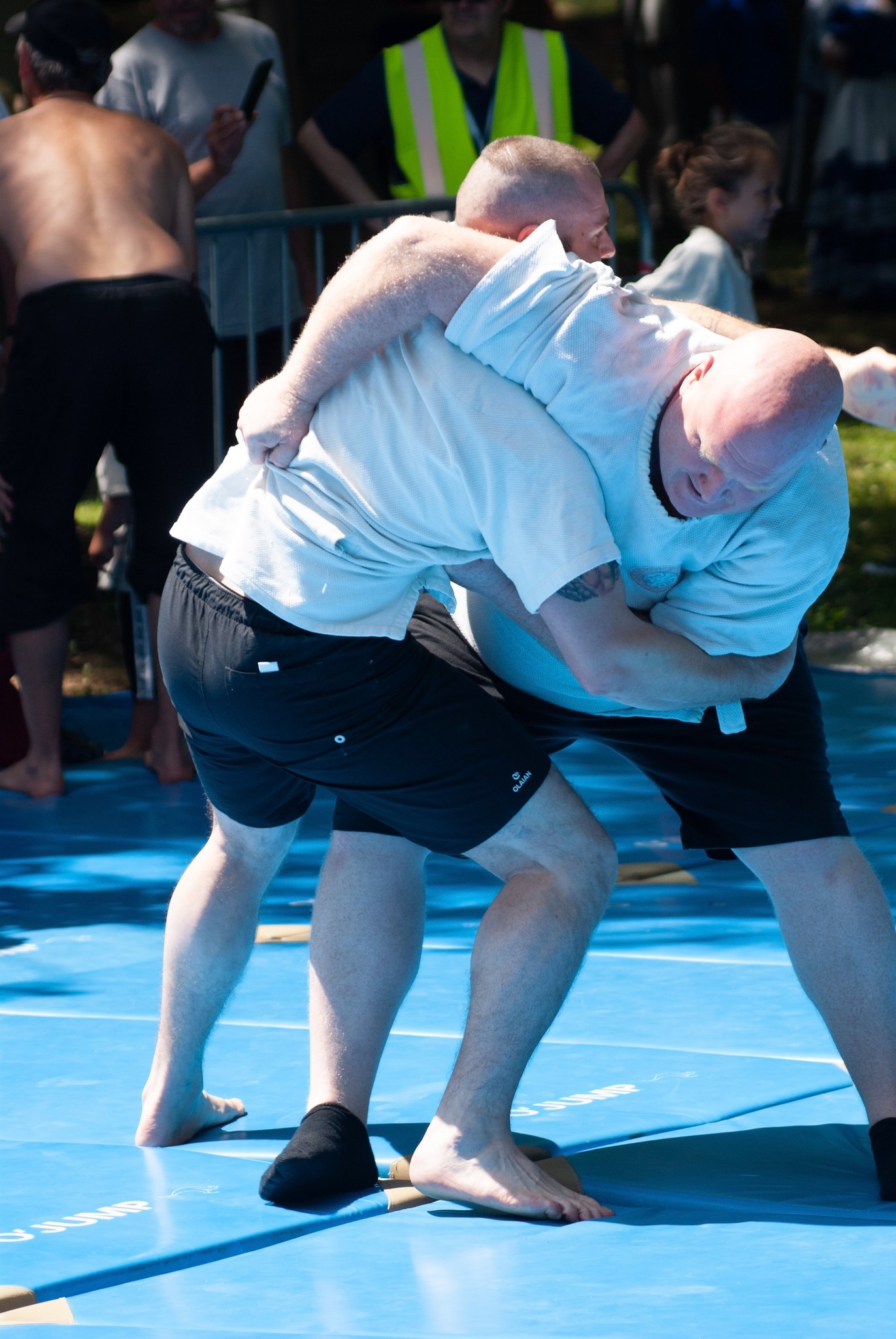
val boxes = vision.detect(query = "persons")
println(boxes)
[648,0,808,299]
[815,1,896,311]
[295,0,647,232]
[626,122,788,327]
[131,138,896,1227]
[0,0,219,797]
[110,0,316,457]
[235,212,895,1214]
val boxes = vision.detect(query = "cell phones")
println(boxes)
[238,59,274,123]
